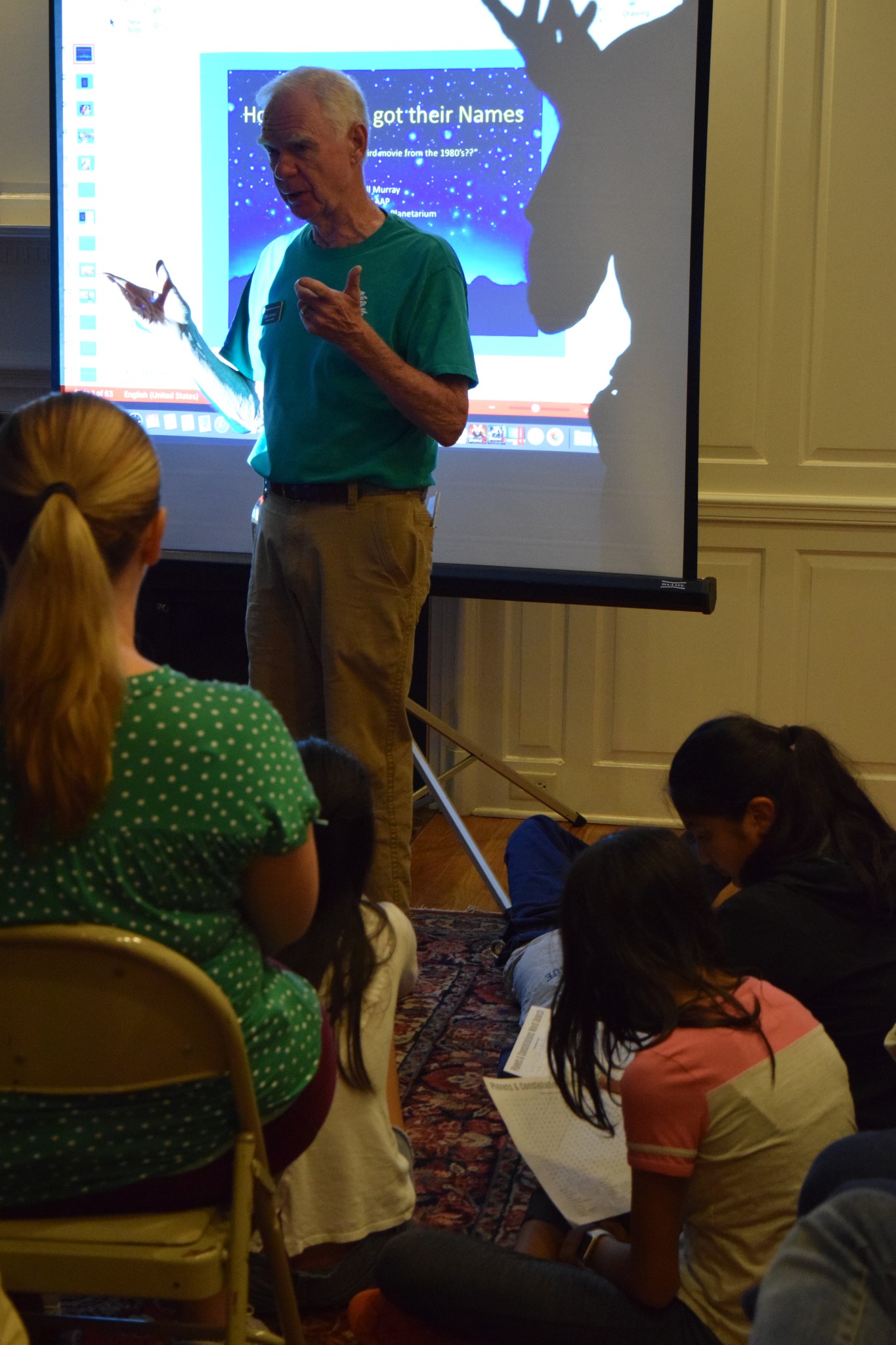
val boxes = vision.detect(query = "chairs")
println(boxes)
[0,922,311,1345]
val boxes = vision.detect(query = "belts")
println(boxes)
[268,475,376,501]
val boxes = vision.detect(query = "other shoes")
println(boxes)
[179,1304,284,1345]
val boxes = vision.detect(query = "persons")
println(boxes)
[246,738,423,1320]
[0,387,338,1218]
[103,66,478,921]
[348,828,857,1345]
[666,714,896,1132]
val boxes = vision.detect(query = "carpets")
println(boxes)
[66,904,632,1345]
